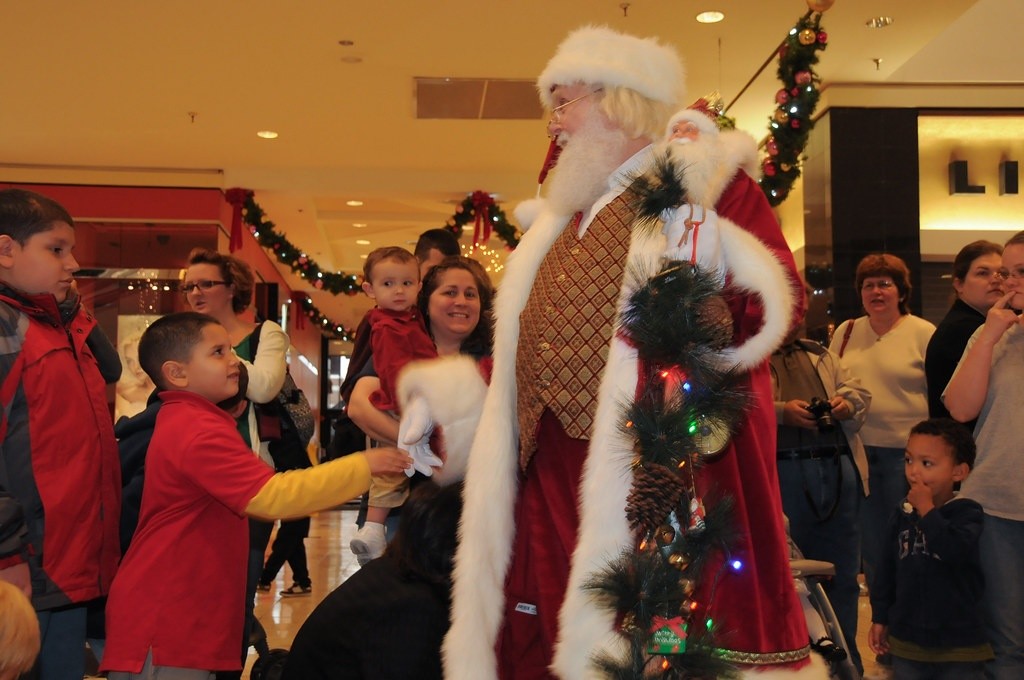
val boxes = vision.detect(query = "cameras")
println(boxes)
[804,397,835,432]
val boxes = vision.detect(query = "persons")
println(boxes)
[281,229,496,679]
[257,514,311,596]
[100,313,414,680]
[178,247,289,655]
[771,283,871,680]
[0,189,122,679]
[442,26,830,680]
[868,420,995,680]
[825,253,936,664]
[924,231,1024,680]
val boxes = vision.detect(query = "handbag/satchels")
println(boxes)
[253,389,314,443]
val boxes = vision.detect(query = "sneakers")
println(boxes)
[257,584,270,594]
[279,583,312,597]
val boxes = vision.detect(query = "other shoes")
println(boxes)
[810,635,847,661]
[350,525,387,567]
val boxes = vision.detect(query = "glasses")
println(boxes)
[860,279,897,289]
[550,88,604,123]
[1000,269,1024,278]
[178,280,233,293]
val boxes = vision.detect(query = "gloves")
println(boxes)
[663,204,726,290]
[397,397,444,478]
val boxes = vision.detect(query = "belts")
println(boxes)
[785,446,829,461]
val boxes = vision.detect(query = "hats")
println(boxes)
[537,25,683,116]
